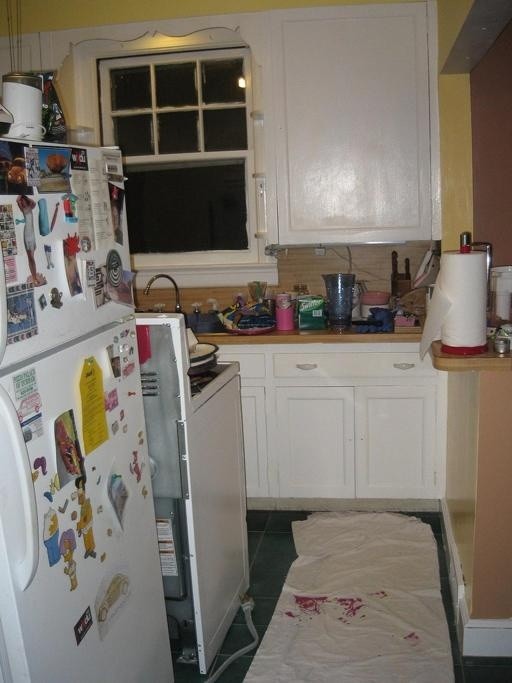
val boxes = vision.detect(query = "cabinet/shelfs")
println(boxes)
[272,345,448,511]
[216,344,268,511]
[269,1,441,247]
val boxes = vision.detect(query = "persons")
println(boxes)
[111,205,121,244]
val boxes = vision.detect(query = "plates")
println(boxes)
[227,325,275,334]
[189,342,219,364]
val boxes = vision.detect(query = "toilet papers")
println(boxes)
[420,252,487,360]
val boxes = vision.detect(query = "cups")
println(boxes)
[321,272,355,329]
[275,305,294,330]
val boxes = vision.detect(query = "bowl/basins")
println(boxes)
[359,290,390,304]
[360,303,389,317]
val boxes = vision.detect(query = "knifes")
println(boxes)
[392,250,411,280]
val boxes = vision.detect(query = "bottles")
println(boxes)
[294,282,308,295]
[2,72,45,141]
[493,338,510,354]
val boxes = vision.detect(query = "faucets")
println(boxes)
[143,273,181,312]
[207,299,219,311]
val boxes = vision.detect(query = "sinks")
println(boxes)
[185,311,225,332]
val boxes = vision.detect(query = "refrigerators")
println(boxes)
[1,134,177,683]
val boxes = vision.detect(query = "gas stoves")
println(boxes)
[179,359,239,421]
